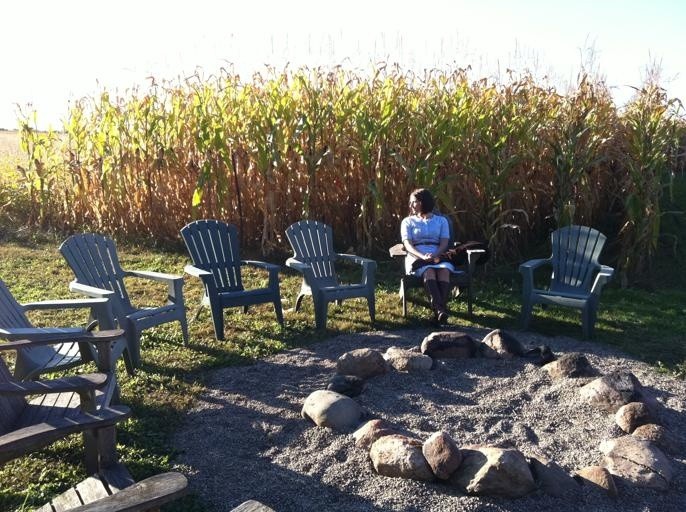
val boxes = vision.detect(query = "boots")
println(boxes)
[422,278,449,325]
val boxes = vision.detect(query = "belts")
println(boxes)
[411,241,441,247]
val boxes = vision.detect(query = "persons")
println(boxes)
[398,186,459,326]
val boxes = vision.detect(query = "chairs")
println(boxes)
[518,225,614,340]
[388,239,485,319]
[58,232,190,366]
[1,405,188,512]
[180,219,283,340]
[1,321,128,480]
[284,219,379,328]
[1,282,132,401]
